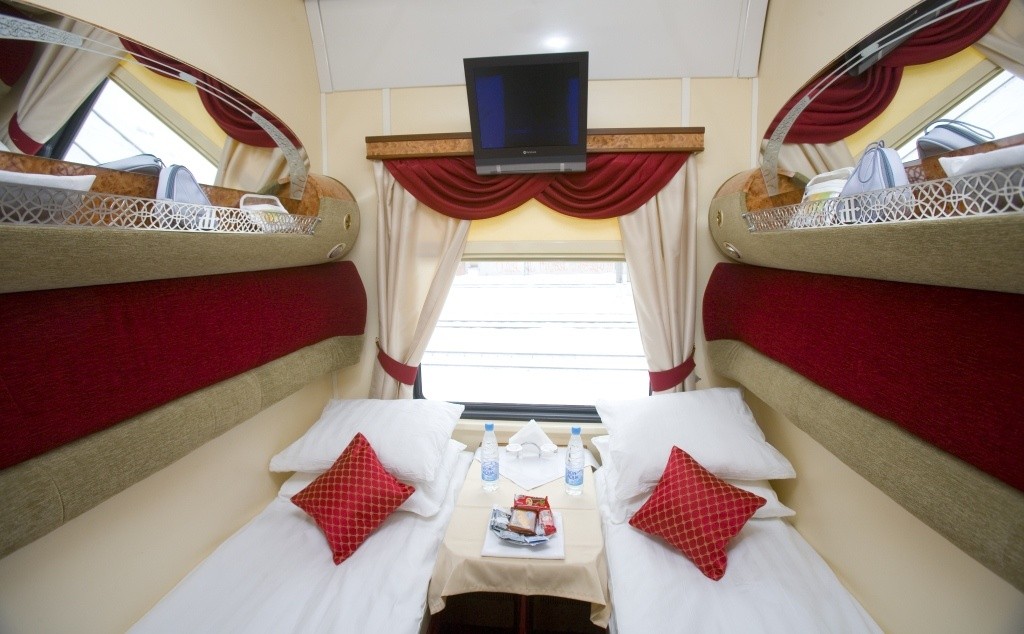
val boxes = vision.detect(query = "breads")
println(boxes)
[508,508,537,534]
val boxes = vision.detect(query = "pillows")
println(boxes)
[290,431,415,566]
[588,435,796,524]
[267,396,466,483]
[277,439,466,520]
[629,445,768,583]
[939,143,1024,213]
[593,388,798,502]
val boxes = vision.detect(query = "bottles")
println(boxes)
[565,425,584,496]
[481,423,499,494]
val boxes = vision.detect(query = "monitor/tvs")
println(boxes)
[464,51,589,173]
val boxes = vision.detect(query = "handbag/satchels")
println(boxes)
[95,154,166,177]
[788,167,855,227]
[240,194,301,233]
[156,164,218,230]
[916,119,996,159]
[836,139,916,222]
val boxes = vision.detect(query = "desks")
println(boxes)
[424,443,613,629]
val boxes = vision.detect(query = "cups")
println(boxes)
[505,444,523,470]
[539,443,558,474]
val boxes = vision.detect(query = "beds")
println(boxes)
[589,465,880,634]
[115,449,473,634]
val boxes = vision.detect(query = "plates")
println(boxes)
[492,511,552,545]
